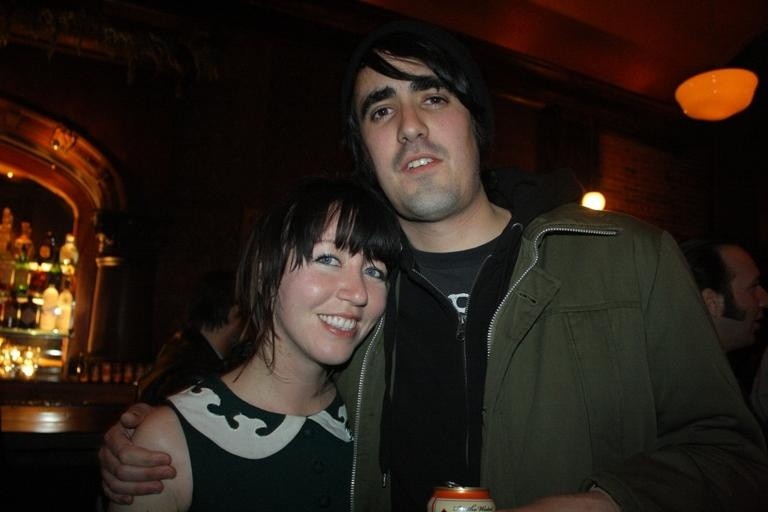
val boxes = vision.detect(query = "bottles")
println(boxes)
[80,362,152,384]
[0,206,78,382]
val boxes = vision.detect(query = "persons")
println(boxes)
[133,267,255,412]
[90,27,767,511]
[111,176,407,511]
[679,233,767,424]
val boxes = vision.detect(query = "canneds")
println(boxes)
[426,480,497,512]
